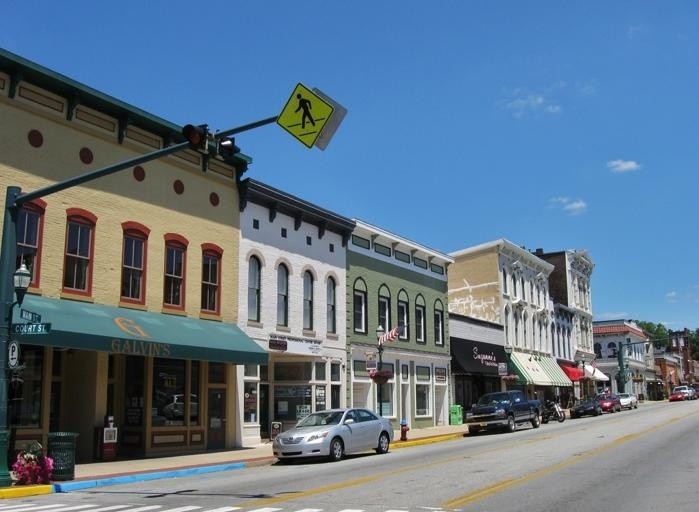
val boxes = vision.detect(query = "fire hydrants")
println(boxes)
[399,417,410,441]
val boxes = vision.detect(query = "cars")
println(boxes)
[272,407,395,462]
[570,398,603,419]
[668,393,688,401]
[595,392,621,413]
[164,393,200,419]
[616,392,638,410]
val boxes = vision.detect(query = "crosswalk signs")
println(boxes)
[277,83,334,149]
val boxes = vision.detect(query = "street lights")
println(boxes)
[377,324,385,414]
[580,353,586,400]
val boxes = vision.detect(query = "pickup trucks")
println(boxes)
[462,388,542,434]
[673,385,699,399]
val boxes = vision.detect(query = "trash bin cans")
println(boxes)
[638,394,644,402]
[450,404,463,425]
[46,432,80,481]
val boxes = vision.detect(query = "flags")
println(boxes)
[590,359,597,370]
[378,324,407,346]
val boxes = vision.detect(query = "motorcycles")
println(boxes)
[542,396,565,423]
